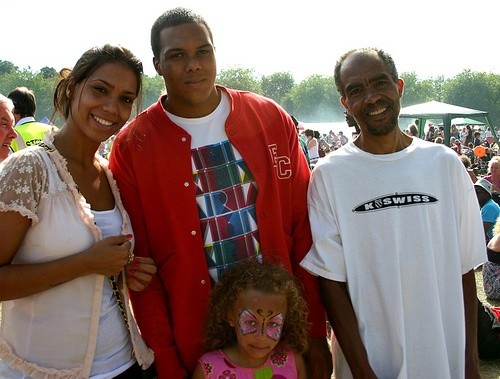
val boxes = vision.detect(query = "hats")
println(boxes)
[474,178,493,197]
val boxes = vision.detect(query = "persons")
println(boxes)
[297,45,488,379]
[101,5,333,379]
[285,108,500,379]
[0,44,160,379]
[191,258,311,379]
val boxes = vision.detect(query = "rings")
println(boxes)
[127,251,135,264]
